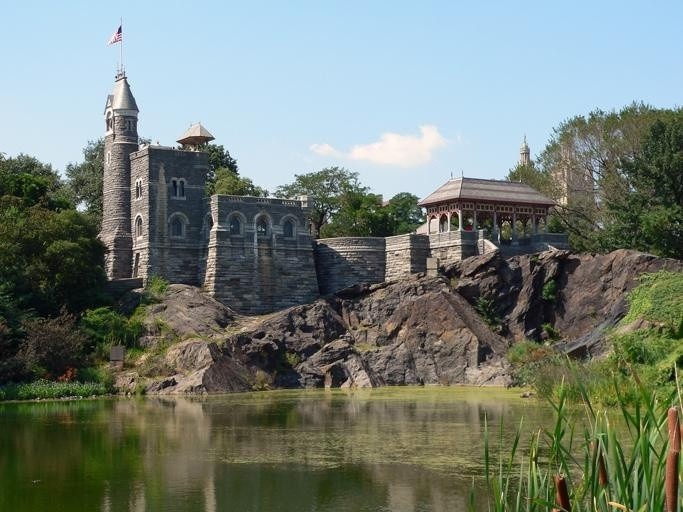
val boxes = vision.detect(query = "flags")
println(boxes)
[107,25,122,45]
